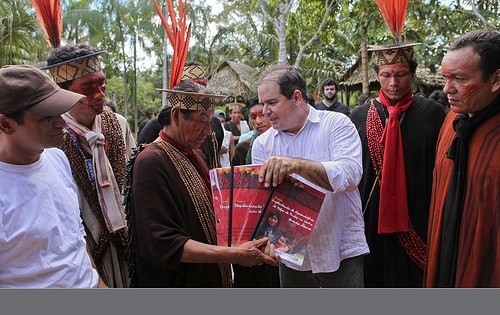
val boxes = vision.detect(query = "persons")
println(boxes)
[348,44,446,288]
[250,67,370,289]
[311,78,350,113]
[422,30,500,289]
[127,61,281,290]
[32,44,139,289]
[0,64,108,289]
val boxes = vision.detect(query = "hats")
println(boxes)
[214,110,226,119]
[0,64,87,116]
[40,51,106,84]
[155,88,227,111]
[224,103,246,110]
[366,43,421,67]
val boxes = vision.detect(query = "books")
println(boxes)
[228,165,272,248]
[250,171,328,266]
[209,167,234,245]
